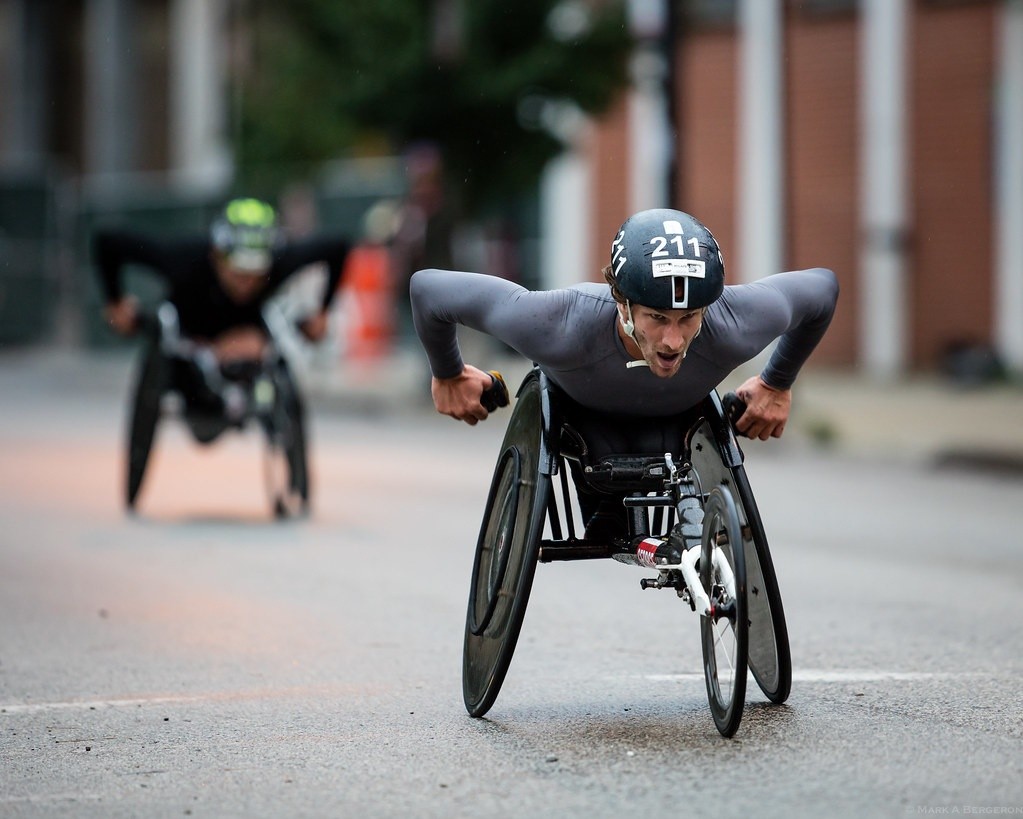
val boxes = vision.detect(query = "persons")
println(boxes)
[411,209,841,496]
[88,193,350,443]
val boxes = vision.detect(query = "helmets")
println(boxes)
[211,199,288,276]
[611,208,726,311]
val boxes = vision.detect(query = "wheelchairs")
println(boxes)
[123,296,311,519]
[461,361,794,739]
